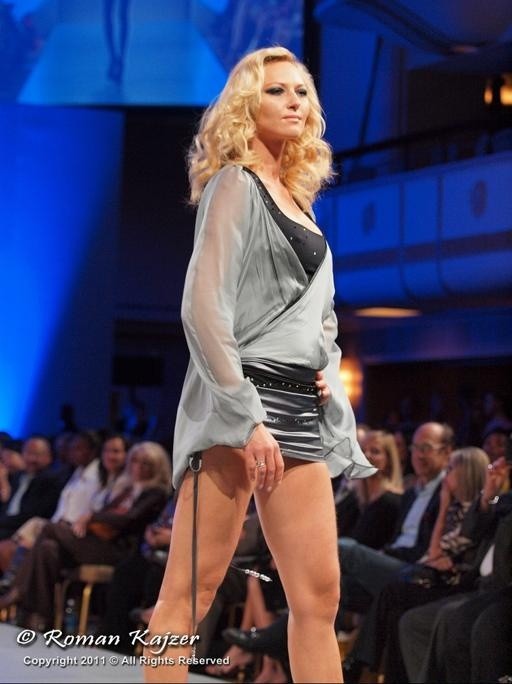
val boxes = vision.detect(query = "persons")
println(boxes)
[1,418,511,684]
[142,44,381,684]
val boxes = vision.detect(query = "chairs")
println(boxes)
[51,563,116,642]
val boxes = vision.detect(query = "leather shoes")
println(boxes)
[221,625,288,664]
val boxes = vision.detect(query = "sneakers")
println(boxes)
[0,581,39,608]
